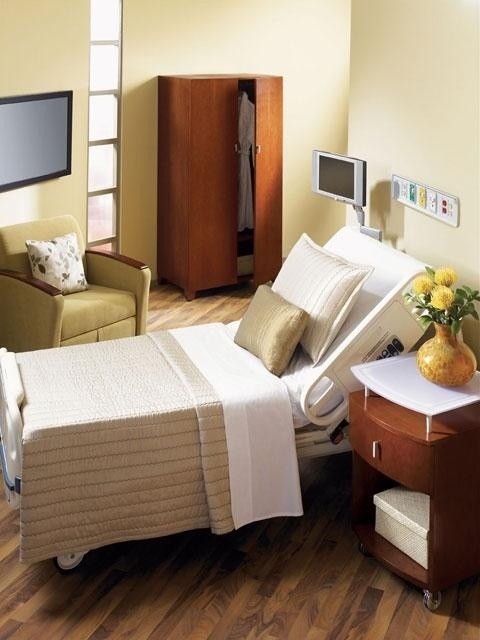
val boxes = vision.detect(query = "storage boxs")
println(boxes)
[373,487,426,571]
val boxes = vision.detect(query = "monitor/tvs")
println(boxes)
[311,150,367,206]
[0,90,73,193]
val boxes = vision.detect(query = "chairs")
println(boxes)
[2,216,155,351]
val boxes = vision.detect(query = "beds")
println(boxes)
[1,227,441,447]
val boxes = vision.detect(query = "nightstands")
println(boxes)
[340,389,479,614]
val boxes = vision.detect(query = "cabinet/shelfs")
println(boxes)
[155,72,285,302]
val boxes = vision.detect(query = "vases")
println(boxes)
[416,322,479,389]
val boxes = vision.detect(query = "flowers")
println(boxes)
[400,262,479,336]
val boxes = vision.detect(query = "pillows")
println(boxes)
[23,233,91,295]
[233,231,377,378]
